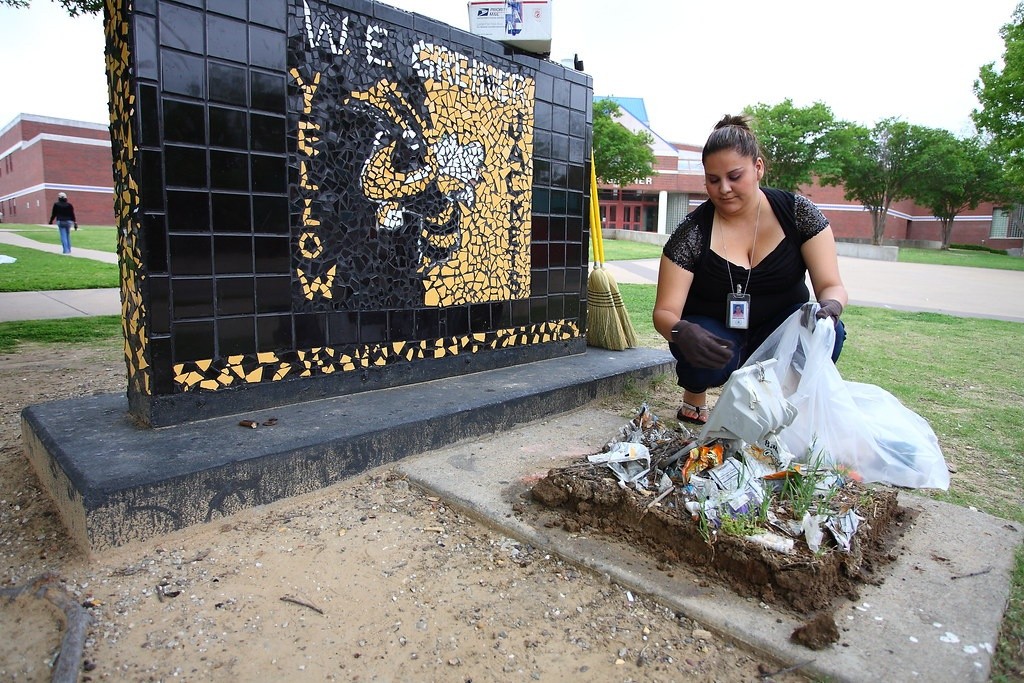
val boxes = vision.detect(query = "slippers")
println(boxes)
[676,401,707,425]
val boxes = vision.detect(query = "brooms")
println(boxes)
[589,150,638,351]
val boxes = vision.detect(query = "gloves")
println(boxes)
[671,320,735,370]
[49,220,52,224]
[800,299,843,334]
[74,223,78,230]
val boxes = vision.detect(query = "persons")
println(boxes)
[0,211,3,223]
[653,114,849,424]
[734,305,743,318]
[49,192,78,254]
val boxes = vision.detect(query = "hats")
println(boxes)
[58,192,66,198]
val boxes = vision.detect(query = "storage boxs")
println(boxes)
[467,0,552,58]
